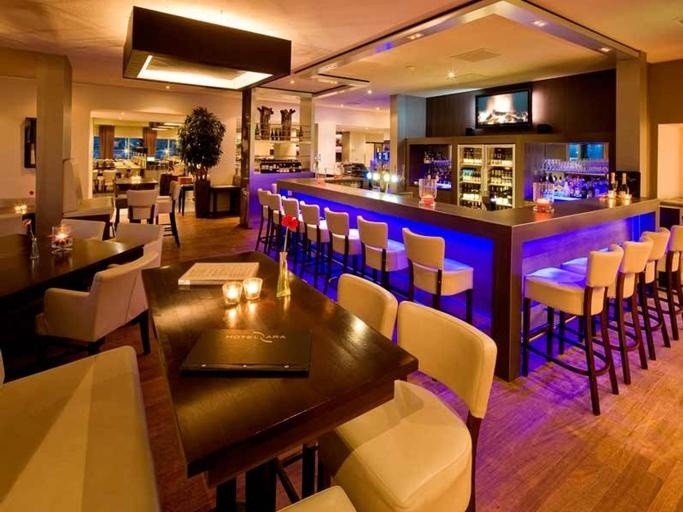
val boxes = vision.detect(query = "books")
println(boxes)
[177,262,260,286]
[181,326,313,376]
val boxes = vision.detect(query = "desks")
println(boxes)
[169,181,194,216]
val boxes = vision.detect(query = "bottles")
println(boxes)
[618,172,630,206]
[459,149,512,209]
[539,159,608,172]
[608,173,617,208]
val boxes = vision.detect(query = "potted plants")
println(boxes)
[179,106,226,218]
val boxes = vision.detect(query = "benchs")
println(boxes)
[35,252,160,356]
[0,346,161,512]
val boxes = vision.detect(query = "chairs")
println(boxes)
[105,221,164,254]
[522,243,624,416]
[402,227,474,322]
[155,183,181,248]
[657,225,683,340]
[281,199,306,244]
[315,300,498,511]
[324,207,362,273]
[299,200,330,260]
[155,180,177,225]
[126,190,158,224]
[592,226,671,360]
[61,219,106,241]
[255,188,271,252]
[335,273,399,339]
[356,215,409,291]
[558,235,656,384]
[267,194,284,250]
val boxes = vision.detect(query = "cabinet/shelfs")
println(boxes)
[457,143,485,210]
[484,142,545,210]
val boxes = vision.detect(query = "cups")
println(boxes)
[242,277,262,300]
[221,282,241,306]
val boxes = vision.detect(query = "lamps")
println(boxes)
[122,5,293,93]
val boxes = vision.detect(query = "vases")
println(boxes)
[30,238,39,259]
[276,251,291,297]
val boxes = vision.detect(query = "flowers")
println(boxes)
[22,219,34,237]
[282,214,298,251]
